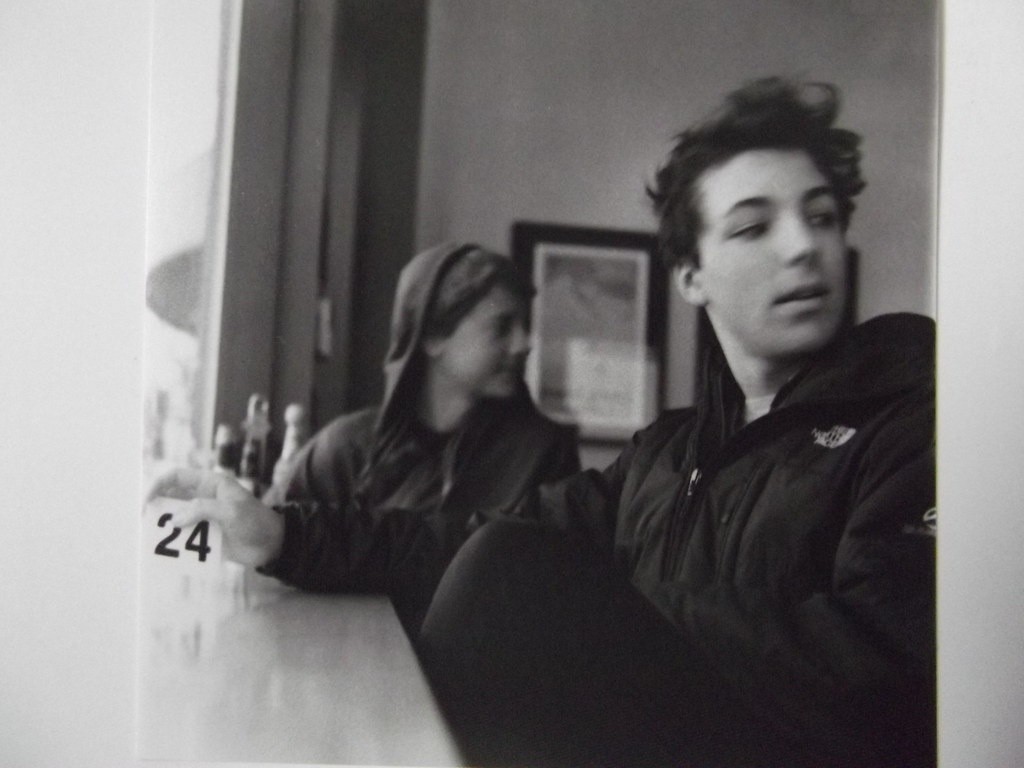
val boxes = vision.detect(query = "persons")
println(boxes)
[265,240,578,516]
[146,72,938,768]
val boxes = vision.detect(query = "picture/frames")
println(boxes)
[508,220,672,445]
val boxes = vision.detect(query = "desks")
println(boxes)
[0,476,467,768]
